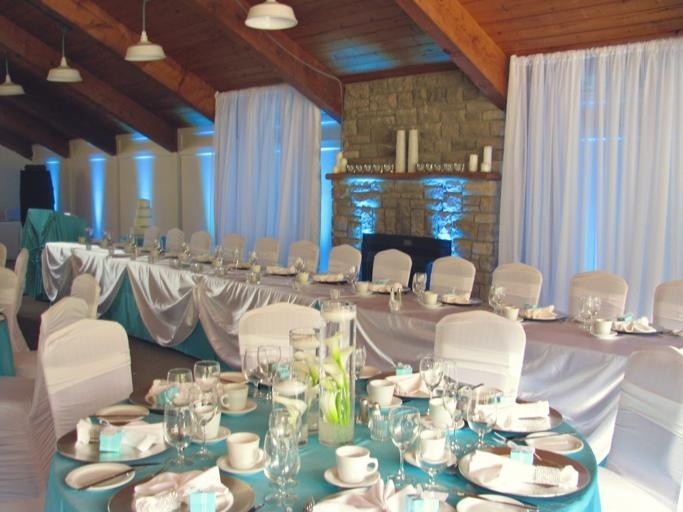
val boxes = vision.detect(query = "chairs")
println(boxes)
[190,230,211,253]
[70,273,100,317]
[430,255,476,297]
[44,318,134,444]
[598,345,683,512]
[1,267,19,377]
[328,243,362,275]
[255,238,280,265]
[288,241,319,273]
[569,270,629,319]
[238,301,328,370]
[433,309,526,399]
[1,296,89,504]
[654,279,682,332]
[221,233,244,260]
[165,228,186,251]
[143,226,161,247]
[19,207,82,300]
[14,247,30,352]
[372,249,412,286]
[491,262,544,307]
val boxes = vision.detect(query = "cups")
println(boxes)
[226,431,262,469]
[596,319,612,334]
[331,289,340,299]
[108,247,303,296]
[390,301,399,312]
[370,415,390,442]
[335,444,379,484]
[355,281,370,294]
[270,369,312,446]
[505,307,519,319]
[367,379,395,403]
[221,383,248,410]
[422,290,438,303]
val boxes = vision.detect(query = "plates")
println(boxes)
[515,318,524,323]
[97,404,149,423]
[325,468,380,487]
[64,462,135,492]
[527,431,584,454]
[222,404,257,417]
[359,366,382,379]
[457,492,529,512]
[217,453,271,474]
[590,331,618,337]
[361,397,402,410]
[217,372,249,384]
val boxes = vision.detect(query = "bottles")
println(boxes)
[359,400,382,423]
[388,287,402,308]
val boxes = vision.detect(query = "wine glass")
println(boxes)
[388,356,499,491]
[489,285,505,317]
[243,342,302,507]
[355,342,367,401]
[411,272,427,303]
[578,296,595,328]
[162,360,220,467]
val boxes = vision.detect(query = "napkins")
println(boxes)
[135,465,229,505]
[526,305,558,322]
[327,478,417,512]
[467,448,530,486]
[612,317,656,334]
[473,402,516,432]
[441,293,472,304]
[384,373,431,397]
[121,418,166,453]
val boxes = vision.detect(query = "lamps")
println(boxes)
[244,0,298,30]
[47,27,82,83]
[124,0,167,62]
[0,56,26,96]
